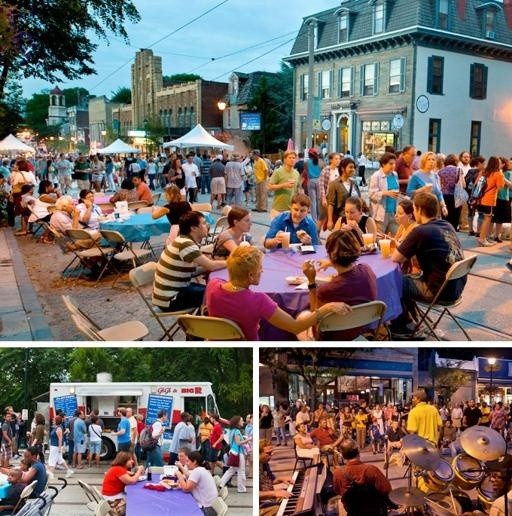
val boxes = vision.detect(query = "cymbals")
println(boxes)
[460,426,506,460]
[402,434,440,470]
[388,486,425,505]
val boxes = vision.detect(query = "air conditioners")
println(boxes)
[484,30,495,38]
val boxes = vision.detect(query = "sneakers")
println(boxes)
[458,225,506,247]
[14,229,27,236]
[208,199,269,214]
[65,469,74,478]
[387,320,419,336]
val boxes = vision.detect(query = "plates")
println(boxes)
[168,483,182,490]
[136,476,148,481]
[286,275,307,285]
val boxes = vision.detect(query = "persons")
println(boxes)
[0,406,252,516]
[259,388,512,516]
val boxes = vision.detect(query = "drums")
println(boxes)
[424,460,453,491]
[421,491,462,515]
[475,473,492,513]
[451,453,482,489]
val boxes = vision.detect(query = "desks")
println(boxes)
[0,482,14,501]
[122,471,205,516]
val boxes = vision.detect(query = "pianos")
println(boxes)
[275,465,319,516]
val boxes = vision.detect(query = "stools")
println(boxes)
[318,449,344,469]
[290,439,314,473]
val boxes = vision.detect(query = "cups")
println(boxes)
[114,212,119,218]
[240,241,249,246]
[280,232,291,251]
[425,182,434,194]
[362,233,376,246]
[379,239,392,259]
[289,180,296,188]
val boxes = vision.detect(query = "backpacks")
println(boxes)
[472,174,488,200]
[138,424,158,450]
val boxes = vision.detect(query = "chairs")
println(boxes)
[208,495,229,516]
[143,466,163,474]
[8,480,41,516]
[76,479,101,511]
[41,469,55,491]
[213,473,223,489]
[1,187,482,341]
[216,485,230,502]
[90,484,114,516]
[93,499,112,516]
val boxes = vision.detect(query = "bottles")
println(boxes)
[2,450,9,468]
[147,463,151,481]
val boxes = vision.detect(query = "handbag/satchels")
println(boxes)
[11,181,28,194]
[453,183,470,209]
[227,451,241,467]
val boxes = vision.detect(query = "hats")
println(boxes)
[21,184,35,196]
